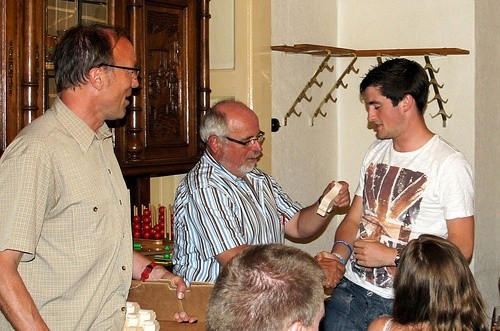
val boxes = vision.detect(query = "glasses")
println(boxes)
[225,130,265,147]
[98,62,141,79]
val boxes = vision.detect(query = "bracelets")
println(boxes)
[332,251,345,267]
[333,240,353,252]
[139,262,159,282]
[394,247,403,268]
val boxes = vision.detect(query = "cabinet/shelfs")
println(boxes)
[0,0,213,181]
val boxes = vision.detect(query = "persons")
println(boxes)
[204,244,327,331]
[316,58,475,331]
[370,234,490,331]
[0,22,198,331]
[173,100,351,289]
